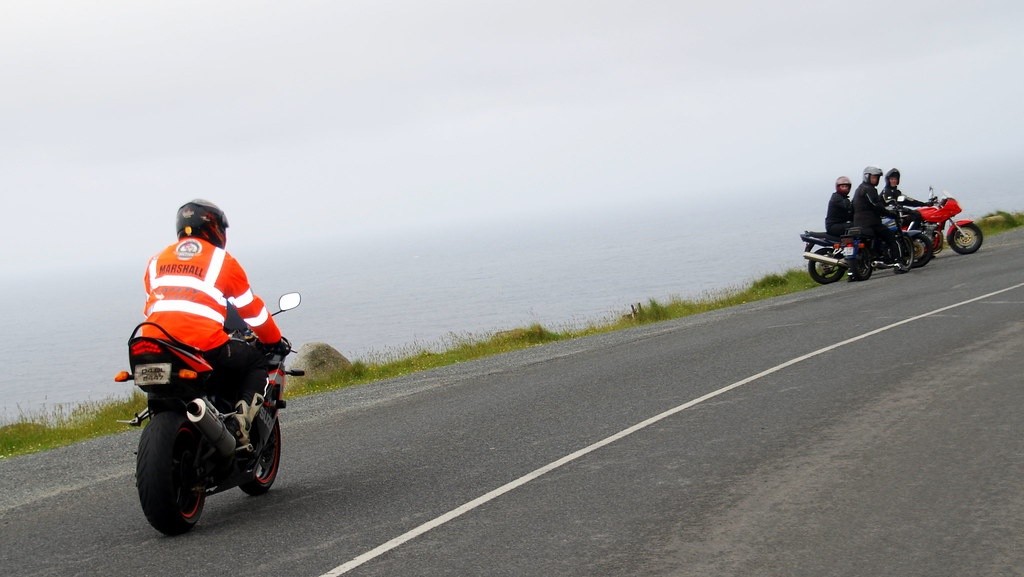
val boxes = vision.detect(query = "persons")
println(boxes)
[825,176,852,235]
[879,168,934,230]
[143,199,281,459]
[847,166,908,282]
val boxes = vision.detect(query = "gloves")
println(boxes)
[908,210,922,217]
[887,210,899,219]
[265,336,293,359]
[924,201,934,207]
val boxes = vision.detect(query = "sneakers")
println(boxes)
[226,415,251,448]
[893,262,910,273]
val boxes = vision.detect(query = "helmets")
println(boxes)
[175,198,229,248]
[835,176,852,198]
[863,166,883,186]
[884,168,900,190]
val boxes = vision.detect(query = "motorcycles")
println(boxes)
[799,184,983,285]
[110,292,307,537]
